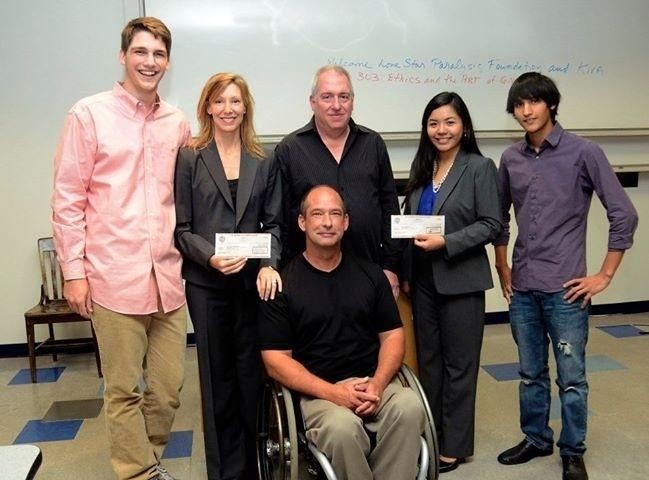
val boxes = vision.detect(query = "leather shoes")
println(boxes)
[562,454,589,480]
[439,457,466,473]
[498,437,554,466]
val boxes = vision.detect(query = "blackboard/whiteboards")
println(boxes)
[138,1,649,145]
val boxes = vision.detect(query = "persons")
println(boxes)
[256,182,424,480]
[493,70,639,479]
[49,18,194,480]
[272,65,404,304]
[171,71,284,480]
[399,90,499,474]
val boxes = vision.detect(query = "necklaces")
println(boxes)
[431,148,458,194]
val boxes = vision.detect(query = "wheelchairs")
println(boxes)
[258,363,441,480]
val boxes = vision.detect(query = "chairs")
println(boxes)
[23,236,102,383]
